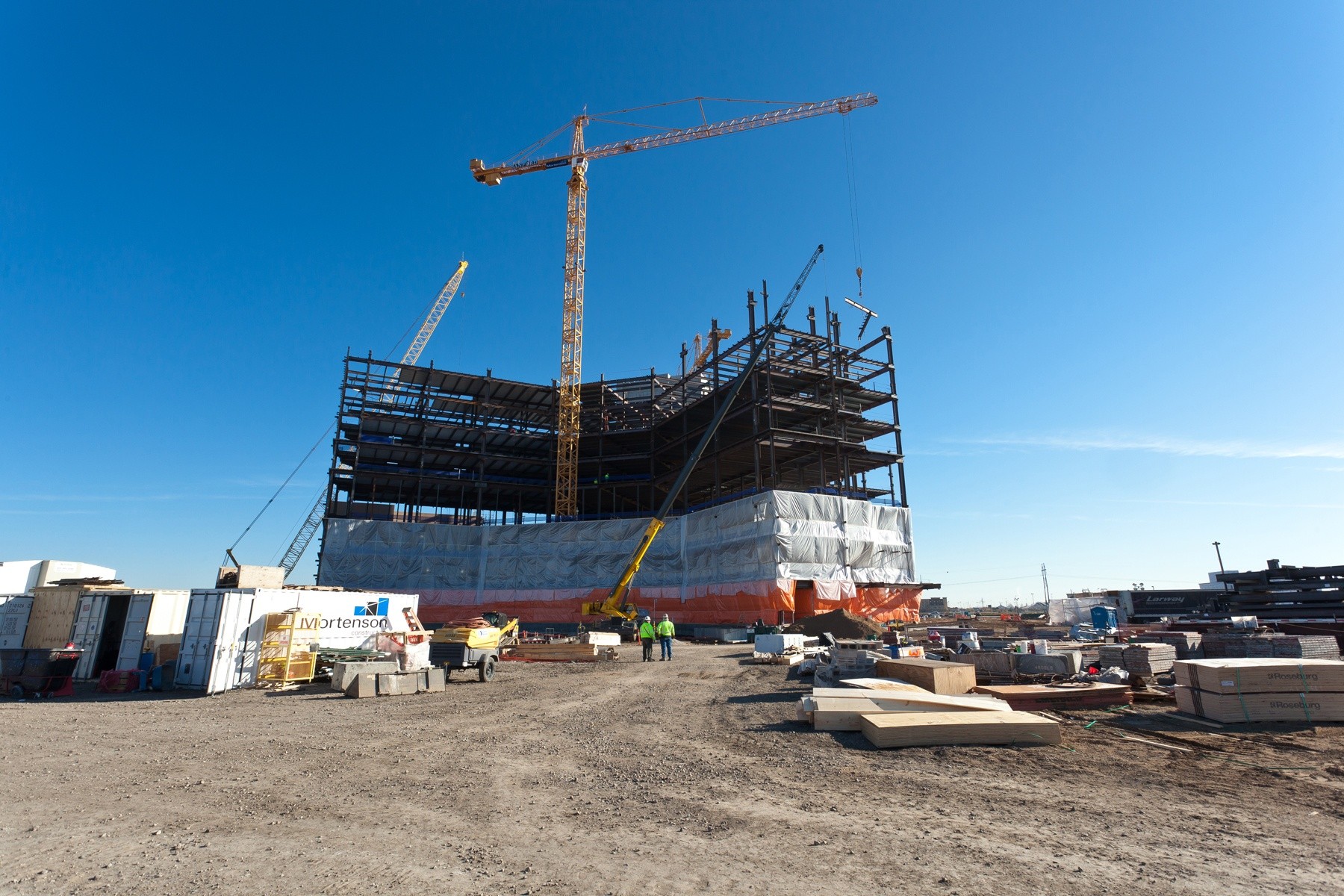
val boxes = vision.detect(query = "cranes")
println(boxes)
[470,92,882,524]
[224,260,468,588]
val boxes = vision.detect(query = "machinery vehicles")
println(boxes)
[581,244,824,642]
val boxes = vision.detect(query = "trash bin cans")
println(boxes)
[139,652,155,671]
[0,649,85,700]
[152,664,170,689]
[166,660,177,681]
[131,672,148,693]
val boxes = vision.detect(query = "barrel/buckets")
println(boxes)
[22,648,55,693]
[0,648,26,683]
[105,651,176,694]
[891,648,900,658]
[1016,639,1048,655]
[1089,667,1099,675]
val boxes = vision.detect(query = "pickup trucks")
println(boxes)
[930,611,945,619]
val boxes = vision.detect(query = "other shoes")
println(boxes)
[668,658,672,660]
[643,659,645,661]
[659,658,665,660]
[648,658,655,661]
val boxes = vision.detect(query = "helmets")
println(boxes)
[663,614,669,618]
[645,616,651,620]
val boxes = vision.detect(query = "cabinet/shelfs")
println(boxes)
[255,612,317,688]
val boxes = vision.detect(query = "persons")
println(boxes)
[656,613,675,662]
[640,616,656,662]
